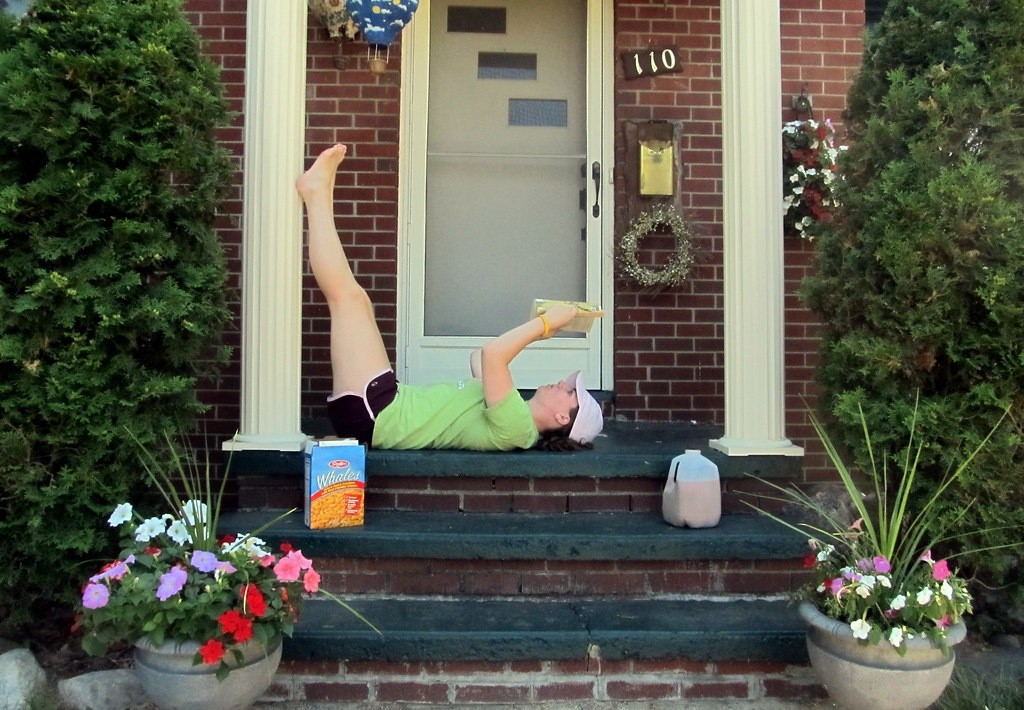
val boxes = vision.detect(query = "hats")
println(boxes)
[568,370,603,445]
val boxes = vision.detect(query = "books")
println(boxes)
[530,299,606,333]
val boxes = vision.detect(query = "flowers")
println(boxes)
[616,204,691,291]
[731,389,1024,658]
[782,118,849,242]
[59,419,383,684]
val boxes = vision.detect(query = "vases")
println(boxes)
[795,599,967,710]
[132,635,282,710]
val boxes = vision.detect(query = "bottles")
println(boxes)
[661,449,722,529]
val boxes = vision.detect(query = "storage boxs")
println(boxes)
[299,435,364,529]
[635,117,674,198]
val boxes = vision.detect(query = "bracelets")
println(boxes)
[538,314,549,338]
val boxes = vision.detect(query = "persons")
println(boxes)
[295,143,604,452]
